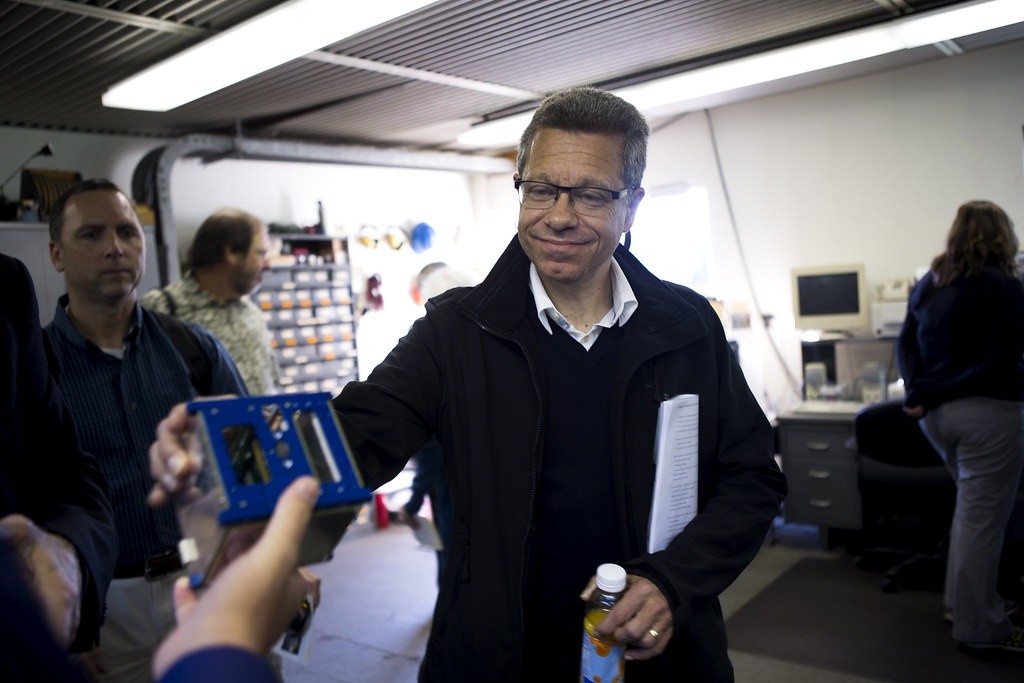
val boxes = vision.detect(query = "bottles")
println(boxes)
[579,563,627,683]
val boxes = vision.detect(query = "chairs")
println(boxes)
[853,401,957,593]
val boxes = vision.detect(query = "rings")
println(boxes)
[647,626,659,638]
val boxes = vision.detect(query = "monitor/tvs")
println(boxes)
[791,262,870,340]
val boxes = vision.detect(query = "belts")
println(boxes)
[112,550,183,582]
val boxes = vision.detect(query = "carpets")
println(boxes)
[723,557,1024,683]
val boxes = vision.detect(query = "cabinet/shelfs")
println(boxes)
[0,222,161,327]
[249,234,360,400]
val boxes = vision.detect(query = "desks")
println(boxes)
[775,412,902,550]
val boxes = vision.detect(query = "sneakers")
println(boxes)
[943,596,1018,621]
[965,625,1024,653]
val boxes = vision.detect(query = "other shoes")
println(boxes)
[397,505,418,529]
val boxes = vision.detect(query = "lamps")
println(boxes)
[0,142,54,189]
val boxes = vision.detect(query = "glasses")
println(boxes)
[513,171,632,217]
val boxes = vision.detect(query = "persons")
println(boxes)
[0,254,118,652]
[897,200,1024,651]
[138,205,277,396]
[144,84,786,683]
[40,178,253,683]
[357,263,447,526]
[0,476,321,683]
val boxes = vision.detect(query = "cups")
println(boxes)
[805,361,826,404]
[861,360,883,404]
